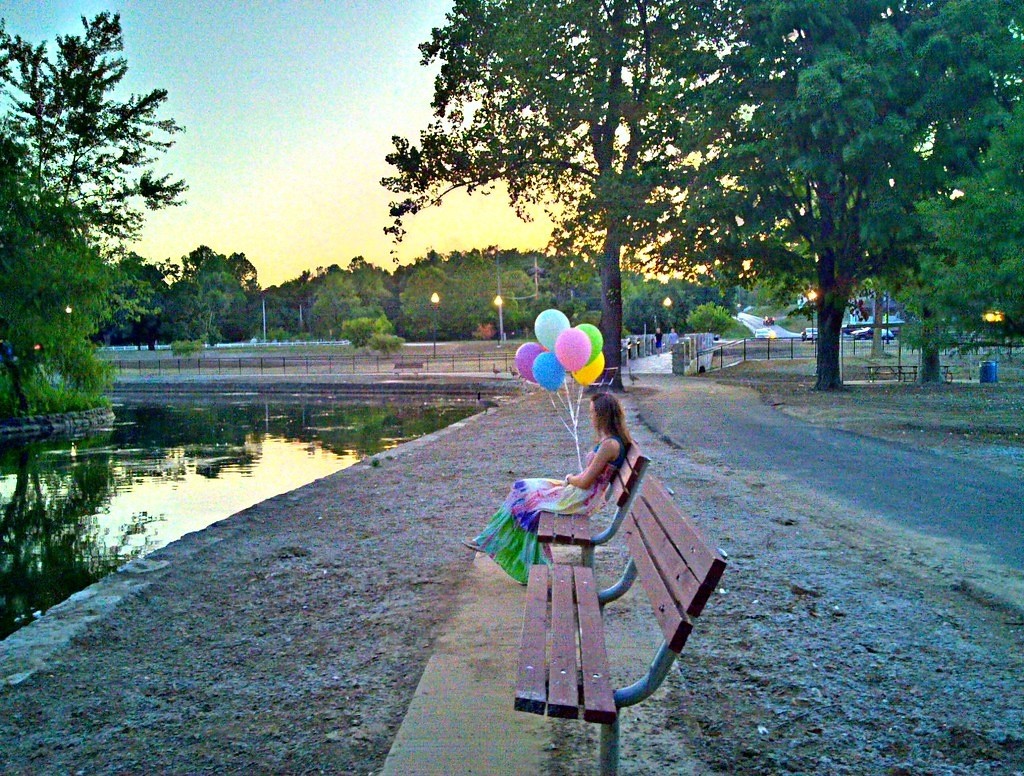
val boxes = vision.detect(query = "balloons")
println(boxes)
[535,309,570,351]
[572,351,605,387]
[555,328,591,371]
[532,353,565,392]
[514,342,545,384]
[575,324,604,367]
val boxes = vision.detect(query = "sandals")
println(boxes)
[462,538,488,553]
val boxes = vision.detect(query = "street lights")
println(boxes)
[663,297,673,334]
[430,292,440,359]
[494,295,504,344]
[808,291,817,344]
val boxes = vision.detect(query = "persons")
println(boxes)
[765,316,768,322]
[461,392,631,584]
[772,316,775,322]
[669,328,679,348]
[654,328,663,357]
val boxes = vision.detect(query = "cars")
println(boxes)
[804,327,818,340]
[850,326,895,341]
[762,317,775,326]
[754,328,778,341]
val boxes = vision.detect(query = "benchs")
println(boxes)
[514,474,729,776]
[537,436,648,567]
[589,367,620,393]
[390,359,423,372]
[866,372,953,382]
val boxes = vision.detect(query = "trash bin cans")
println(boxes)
[979,360,999,383]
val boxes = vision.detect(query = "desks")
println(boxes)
[864,366,950,382]
